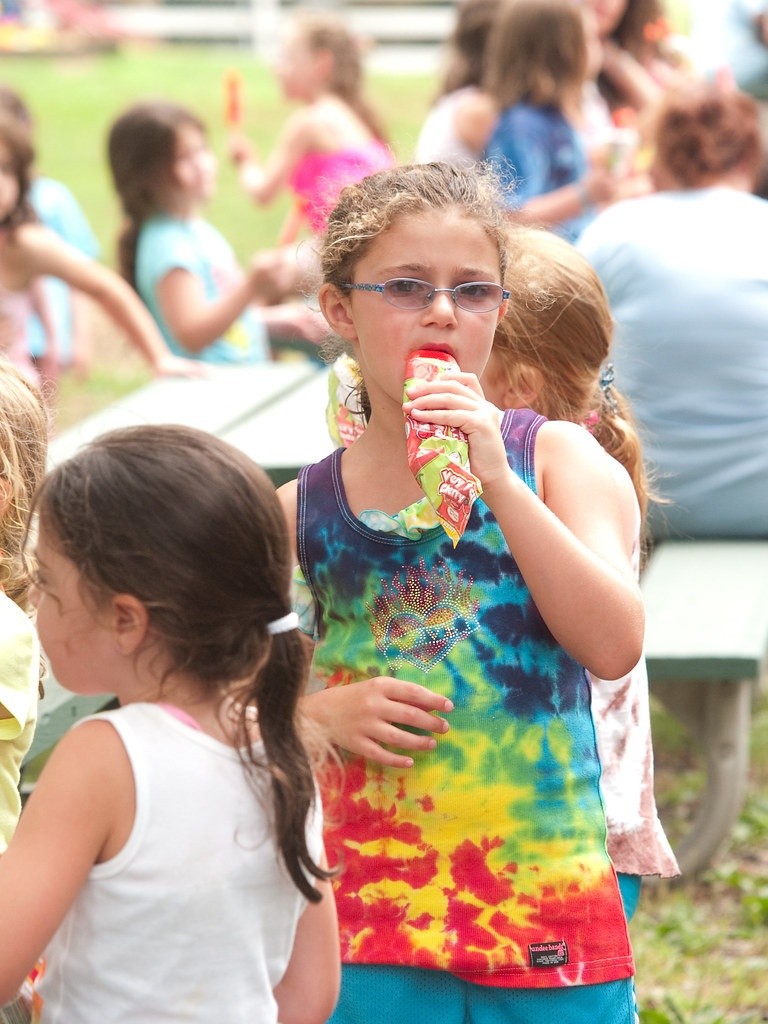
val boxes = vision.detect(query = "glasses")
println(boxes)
[342,278,509,313]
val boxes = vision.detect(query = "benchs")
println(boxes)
[44,358,768,895]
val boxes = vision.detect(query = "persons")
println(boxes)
[105,102,321,365]
[0,111,194,377]
[480,224,680,925]
[277,160,643,1023]
[413,2,499,162]
[0,87,109,369]
[0,424,342,1023]
[480,2,620,243]
[590,0,768,125]
[230,21,394,307]
[573,86,767,537]
[0,357,46,857]
